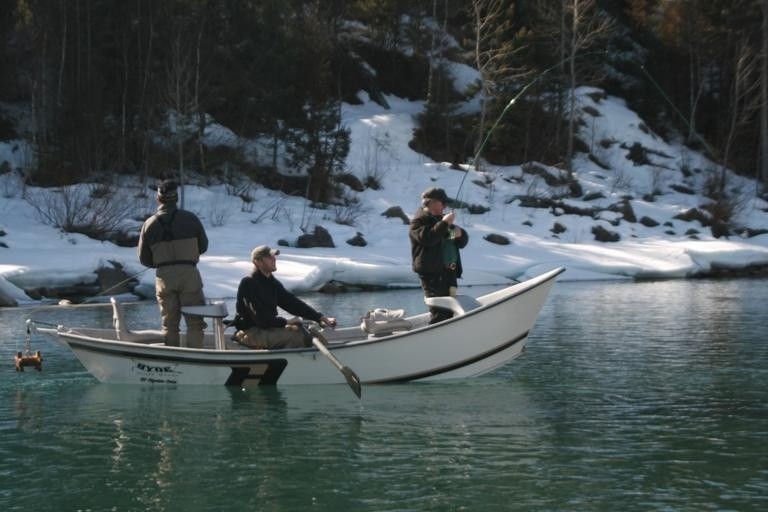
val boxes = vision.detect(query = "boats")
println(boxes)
[16,263,572,390]
[73,381,533,430]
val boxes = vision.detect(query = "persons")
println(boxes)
[233,243,337,350]
[408,187,468,324]
[138,179,208,348]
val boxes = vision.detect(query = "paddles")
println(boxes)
[300,325,362,398]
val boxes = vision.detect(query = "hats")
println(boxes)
[157,180,177,202]
[251,246,279,264]
[422,187,453,204]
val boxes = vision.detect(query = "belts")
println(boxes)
[443,263,456,271]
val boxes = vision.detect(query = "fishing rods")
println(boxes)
[445,50,643,240]
[83,266,153,304]
